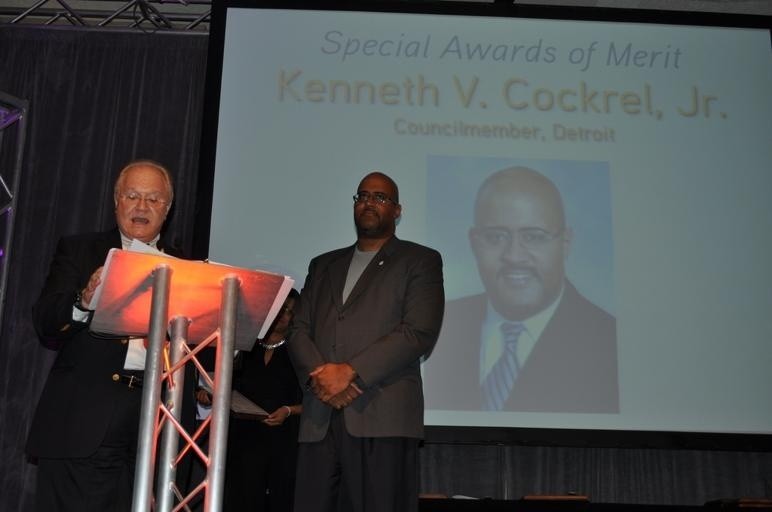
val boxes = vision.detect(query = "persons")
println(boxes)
[420,164,619,413]
[283,170,445,511]
[196,286,307,511]
[24,157,196,511]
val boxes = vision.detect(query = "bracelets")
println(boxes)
[282,404,292,418]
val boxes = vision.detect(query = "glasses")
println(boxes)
[470,227,569,250]
[352,191,398,208]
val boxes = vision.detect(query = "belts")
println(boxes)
[117,372,146,390]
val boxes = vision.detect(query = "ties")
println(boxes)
[479,317,527,413]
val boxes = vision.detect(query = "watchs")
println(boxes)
[73,291,91,313]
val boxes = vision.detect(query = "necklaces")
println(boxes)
[257,337,290,351]
